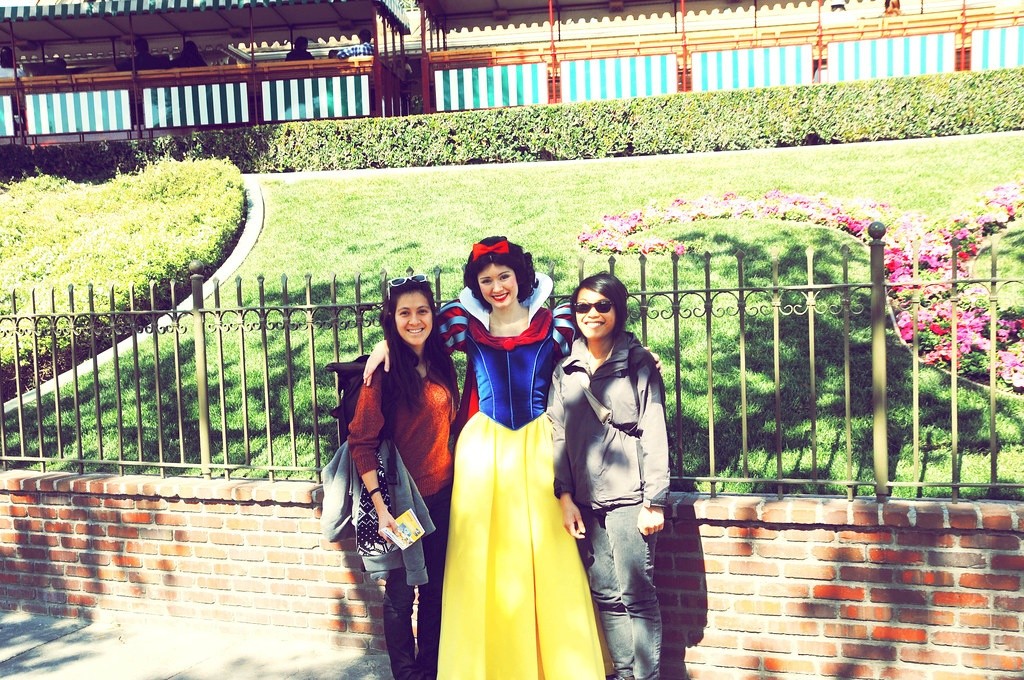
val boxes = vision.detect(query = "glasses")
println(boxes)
[574,300,614,313]
[388,274,428,300]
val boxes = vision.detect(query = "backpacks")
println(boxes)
[325,355,395,449]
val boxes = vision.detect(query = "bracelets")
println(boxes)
[368,488,381,497]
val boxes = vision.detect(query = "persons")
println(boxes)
[285,37,314,62]
[170,41,208,68]
[336,29,374,58]
[86,38,170,74]
[0,46,26,78]
[329,50,339,58]
[546,269,671,680]
[813,5,857,79]
[881,0,904,18]
[363,237,662,680]
[36,59,72,75]
[348,280,460,680]
